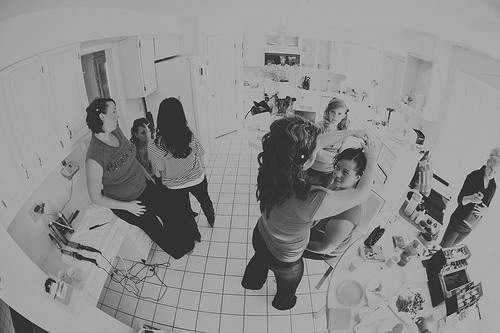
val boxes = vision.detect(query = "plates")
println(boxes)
[336,280,363,307]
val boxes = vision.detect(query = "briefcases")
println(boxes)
[423,244,484,317]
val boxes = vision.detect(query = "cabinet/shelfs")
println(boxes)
[52,205,164,305]
[265,76,419,218]
[145,53,213,167]
[121,33,180,99]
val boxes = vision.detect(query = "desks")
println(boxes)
[390,198,447,241]
[315,231,463,333]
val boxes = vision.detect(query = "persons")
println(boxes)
[416,317,433,333]
[143,97,215,244]
[304,97,350,189]
[84,99,199,259]
[240,116,377,311]
[438,148,500,249]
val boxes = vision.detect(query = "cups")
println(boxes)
[348,262,358,272]
[67,268,82,282]
[58,270,72,284]
[386,253,400,268]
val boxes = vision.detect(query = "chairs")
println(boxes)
[314,189,386,289]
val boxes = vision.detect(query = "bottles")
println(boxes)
[398,240,420,266]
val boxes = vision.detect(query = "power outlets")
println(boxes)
[28,204,44,224]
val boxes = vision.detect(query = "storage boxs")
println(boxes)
[426,244,484,316]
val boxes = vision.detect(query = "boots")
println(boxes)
[200,200,215,226]
[183,209,202,241]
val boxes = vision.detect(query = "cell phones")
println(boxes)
[478,191,484,199]
